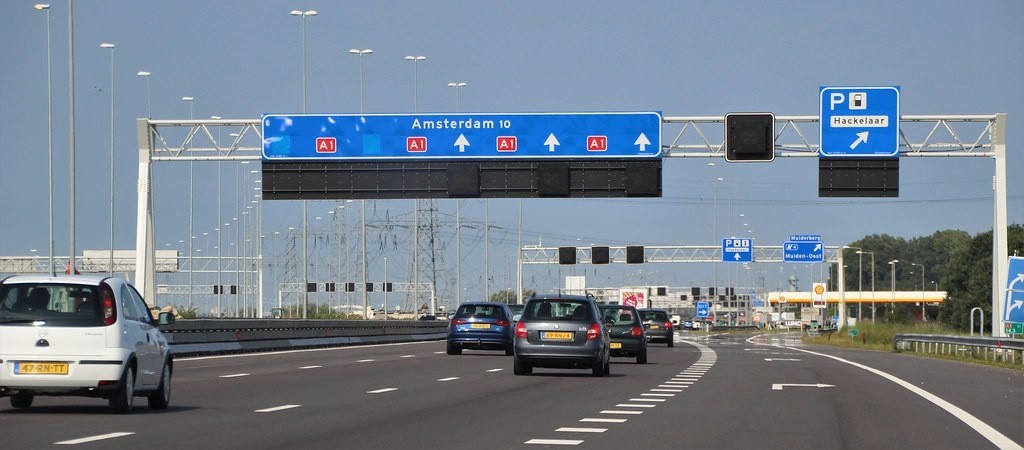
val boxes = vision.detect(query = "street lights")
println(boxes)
[709,163,834,326]
[229,131,241,319]
[888,259,899,314]
[856,251,874,324]
[931,281,938,291]
[210,116,222,319]
[909,271,917,291]
[136,71,157,308]
[911,263,925,321]
[99,43,116,277]
[33,3,55,312]
[348,47,375,321]
[181,95,194,311]
[447,81,467,311]
[290,10,320,320]
[164,161,360,319]
[404,54,427,322]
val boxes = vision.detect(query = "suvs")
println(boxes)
[446,301,518,356]
[599,304,650,364]
[0,271,176,415]
[637,308,678,347]
[513,291,616,376]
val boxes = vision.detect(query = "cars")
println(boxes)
[670,316,714,330]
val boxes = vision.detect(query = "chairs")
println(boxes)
[537,308,551,317]
[656,315,663,320]
[573,306,586,318]
[620,314,631,321]
[466,306,475,317]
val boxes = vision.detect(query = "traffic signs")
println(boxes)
[261,111,663,160]
[696,301,709,317]
[819,86,901,159]
[722,238,753,264]
[783,234,824,264]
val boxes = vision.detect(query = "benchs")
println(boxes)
[12,300,94,313]
